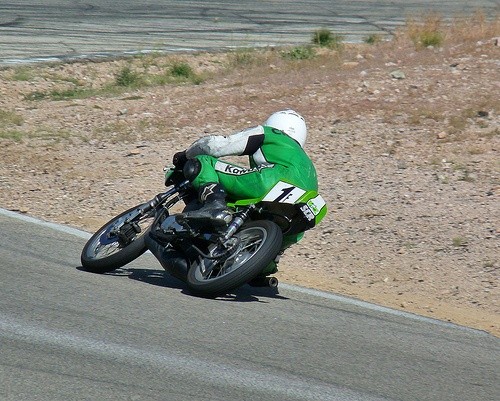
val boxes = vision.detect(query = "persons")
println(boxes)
[164,109,318,276]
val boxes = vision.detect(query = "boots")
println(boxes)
[175,182,232,227]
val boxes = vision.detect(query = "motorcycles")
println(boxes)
[80,151,328,297]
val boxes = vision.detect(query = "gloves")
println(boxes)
[173,152,188,166]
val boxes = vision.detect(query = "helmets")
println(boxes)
[263,109,307,148]
